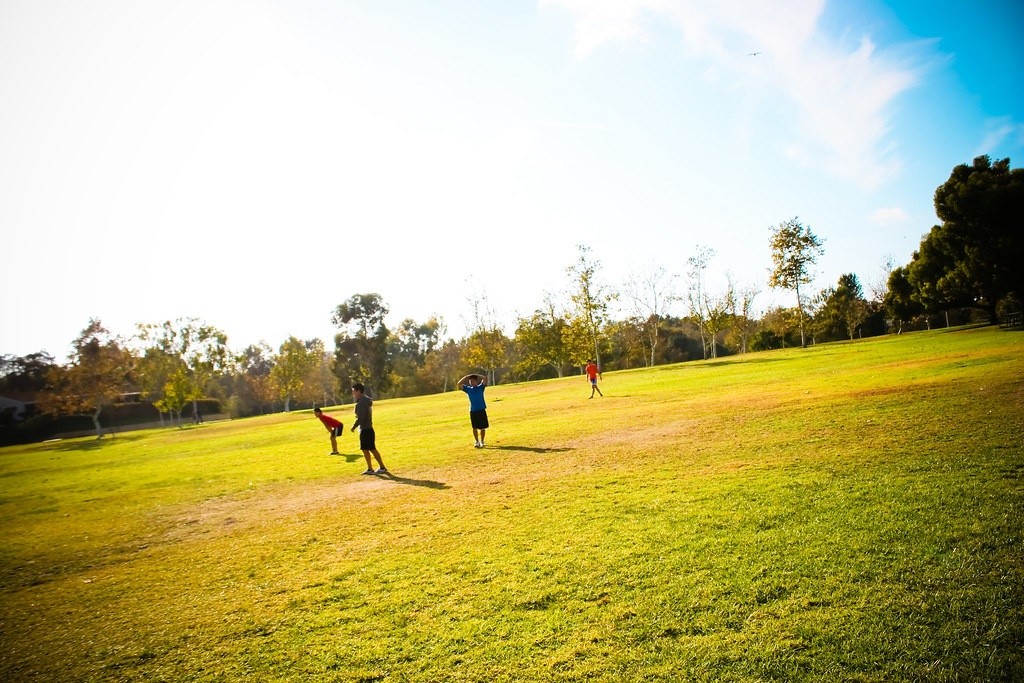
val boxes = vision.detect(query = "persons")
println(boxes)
[456,373,489,448]
[585,358,603,399]
[313,407,344,455]
[350,383,387,475]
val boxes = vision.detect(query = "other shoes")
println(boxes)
[330,452,338,455]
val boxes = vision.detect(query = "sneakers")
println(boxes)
[474,441,483,447]
[363,469,374,474]
[372,468,387,474]
